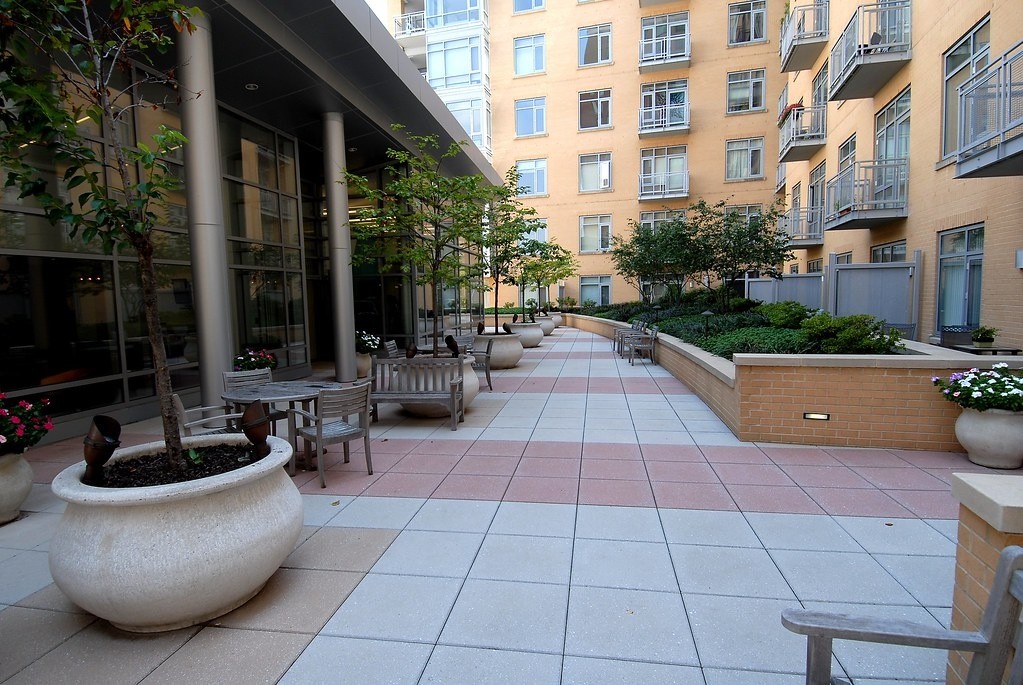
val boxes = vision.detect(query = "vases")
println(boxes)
[955,402,1023,469]
[0,453,33,526]
[354,351,371,378]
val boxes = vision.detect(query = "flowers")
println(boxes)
[233,347,278,370]
[777,104,804,129]
[0,391,56,456]
[355,330,380,354]
[930,362,1023,412]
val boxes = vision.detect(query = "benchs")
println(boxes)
[353,354,464,432]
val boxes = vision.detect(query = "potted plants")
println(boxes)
[0,0,302,634]
[969,325,1000,348]
[833,198,856,215]
[335,122,579,419]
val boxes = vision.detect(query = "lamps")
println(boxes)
[502,321,512,334]
[528,313,535,323]
[444,335,461,358]
[405,342,417,359]
[82,414,121,485]
[239,398,271,461]
[477,321,486,335]
[510,314,518,323]
[541,308,548,316]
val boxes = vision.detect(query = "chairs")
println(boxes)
[221,368,288,437]
[416,342,467,355]
[286,382,373,489]
[383,339,407,358]
[467,339,494,391]
[613,320,659,366]
[171,393,245,438]
